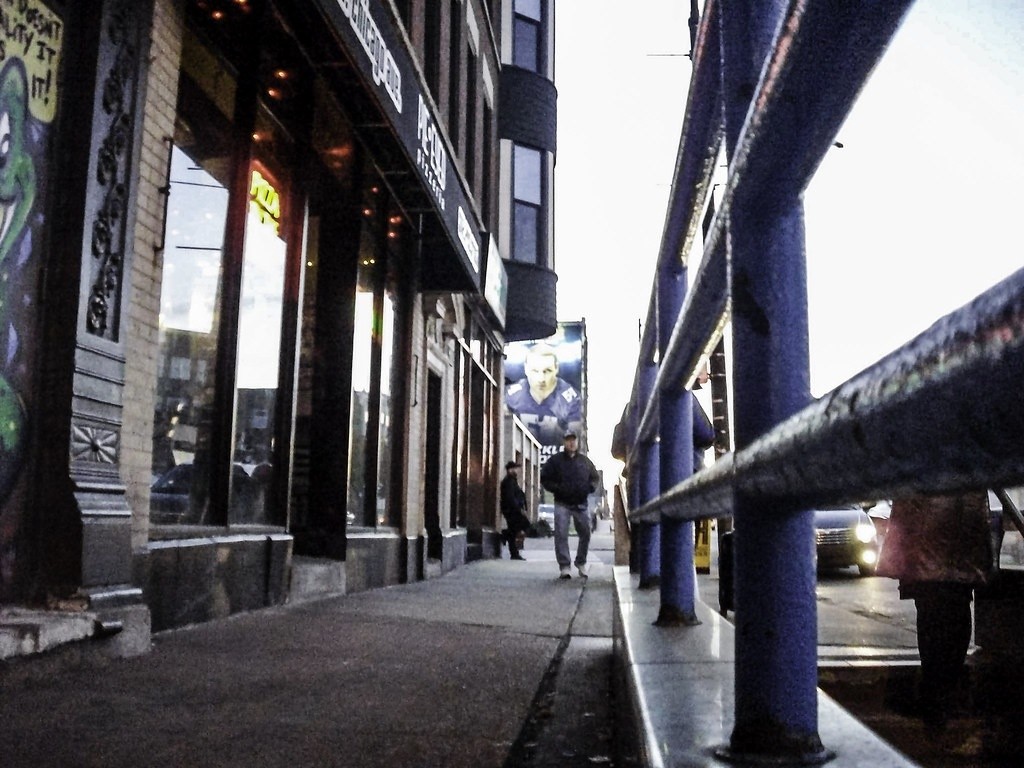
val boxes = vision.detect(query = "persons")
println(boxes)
[504,343,583,431]
[692,370,715,546]
[499,461,532,561]
[875,489,990,755]
[541,429,600,579]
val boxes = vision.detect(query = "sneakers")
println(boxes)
[574,561,588,577]
[559,568,571,579]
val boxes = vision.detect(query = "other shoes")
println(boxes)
[499,533,506,546]
[510,555,526,561]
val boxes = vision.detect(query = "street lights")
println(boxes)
[707,134,866,616]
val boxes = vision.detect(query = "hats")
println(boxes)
[505,461,522,470]
[563,429,577,439]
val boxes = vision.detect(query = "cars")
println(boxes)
[813,503,877,578]
[538,504,557,531]
[151,462,259,525]
